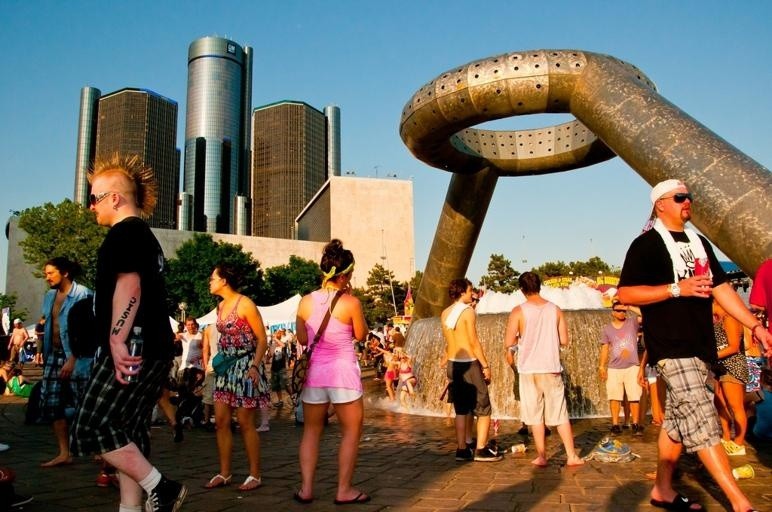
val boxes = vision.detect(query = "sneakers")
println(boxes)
[724,440,746,456]
[145,473,188,512]
[474,447,502,462]
[456,447,475,461]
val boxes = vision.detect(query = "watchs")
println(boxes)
[669,282,682,300]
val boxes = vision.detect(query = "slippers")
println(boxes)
[294,490,312,503]
[650,494,702,512]
[335,493,370,504]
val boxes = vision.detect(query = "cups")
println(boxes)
[694,258,711,293]
[245,378,256,398]
[732,463,754,480]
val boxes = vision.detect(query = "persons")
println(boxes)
[35,315,46,364]
[69,152,191,512]
[200,258,269,491]
[8,318,29,361]
[355,322,416,402]
[713,298,771,455]
[1,360,35,398]
[616,178,772,511]
[39,257,94,472]
[750,258,772,376]
[255,327,303,431]
[148,317,219,442]
[438,277,506,463]
[506,271,589,467]
[599,301,665,434]
[291,238,375,505]
[469,288,480,309]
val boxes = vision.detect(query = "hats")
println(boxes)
[648,179,685,221]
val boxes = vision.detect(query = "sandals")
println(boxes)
[204,475,232,489]
[239,476,262,490]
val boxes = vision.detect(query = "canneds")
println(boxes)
[694,255,710,298]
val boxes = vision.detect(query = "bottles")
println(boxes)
[124,327,144,382]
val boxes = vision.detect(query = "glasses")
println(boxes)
[614,309,626,312]
[472,297,479,302]
[89,193,112,204]
[660,193,693,203]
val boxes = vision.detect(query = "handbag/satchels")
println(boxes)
[212,348,256,376]
[23,380,50,425]
[291,353,310,392]
